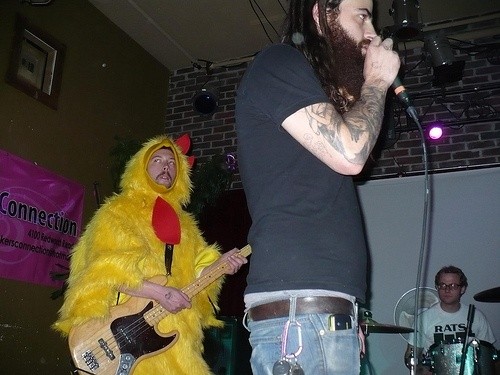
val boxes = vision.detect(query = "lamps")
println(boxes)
[193,78,225,115]
[387,0,424,38]
[422,30,456,68]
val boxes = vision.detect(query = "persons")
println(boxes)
[403,265,496,374]
[52,135,249,375]
[234,0,401,374]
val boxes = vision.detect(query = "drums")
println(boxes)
[428,340,500,375]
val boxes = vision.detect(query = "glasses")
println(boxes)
[437,282,464,290]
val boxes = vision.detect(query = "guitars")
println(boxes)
[68,244,252,375]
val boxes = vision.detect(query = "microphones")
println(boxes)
[392,77,418,119]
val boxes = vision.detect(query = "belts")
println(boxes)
[251,297,355,321]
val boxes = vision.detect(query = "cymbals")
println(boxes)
[473,287,500,303]
[358,320,418,334]
